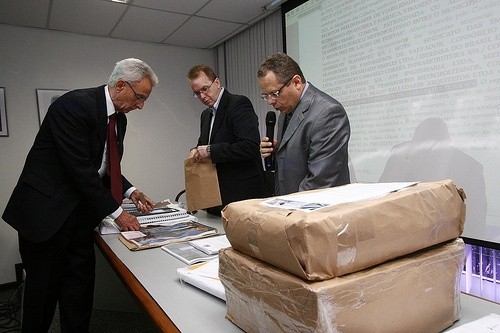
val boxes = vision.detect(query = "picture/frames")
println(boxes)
[35,88,69,127]
[0,86,9,137]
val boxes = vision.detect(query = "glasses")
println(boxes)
[193,78,215,98]
[125,81,147,102]
[261,74,295,101]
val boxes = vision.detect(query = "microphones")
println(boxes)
[264,111,276,170]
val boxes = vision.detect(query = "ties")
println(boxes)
[280,113,291,143]
[109,113,122,205]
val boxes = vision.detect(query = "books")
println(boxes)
[163,234,233,263]
[99,198,217,248]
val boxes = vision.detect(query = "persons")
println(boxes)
[1,58,158,333]
[257,54,351,197]
[189,65,270,219]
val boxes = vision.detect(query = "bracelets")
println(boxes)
[207,145,211,156]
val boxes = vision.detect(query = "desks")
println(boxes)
[91,201,500,333]
